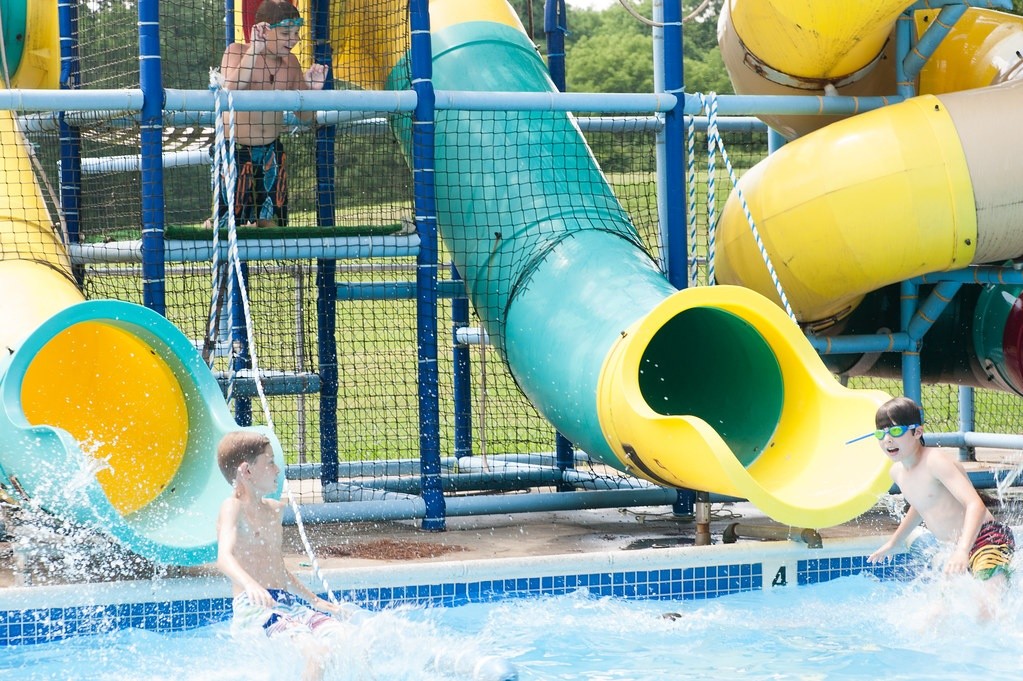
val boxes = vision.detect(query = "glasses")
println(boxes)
[873,424,919,439]
[270,18,304,29]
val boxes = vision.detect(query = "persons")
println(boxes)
[208,0,330,228]
[865,396,1015,620]
[215,431,335,629]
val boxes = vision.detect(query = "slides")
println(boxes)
[236,0,1023,529]
[0,0,284,566]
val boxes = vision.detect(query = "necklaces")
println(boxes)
[260,51,283,84]
